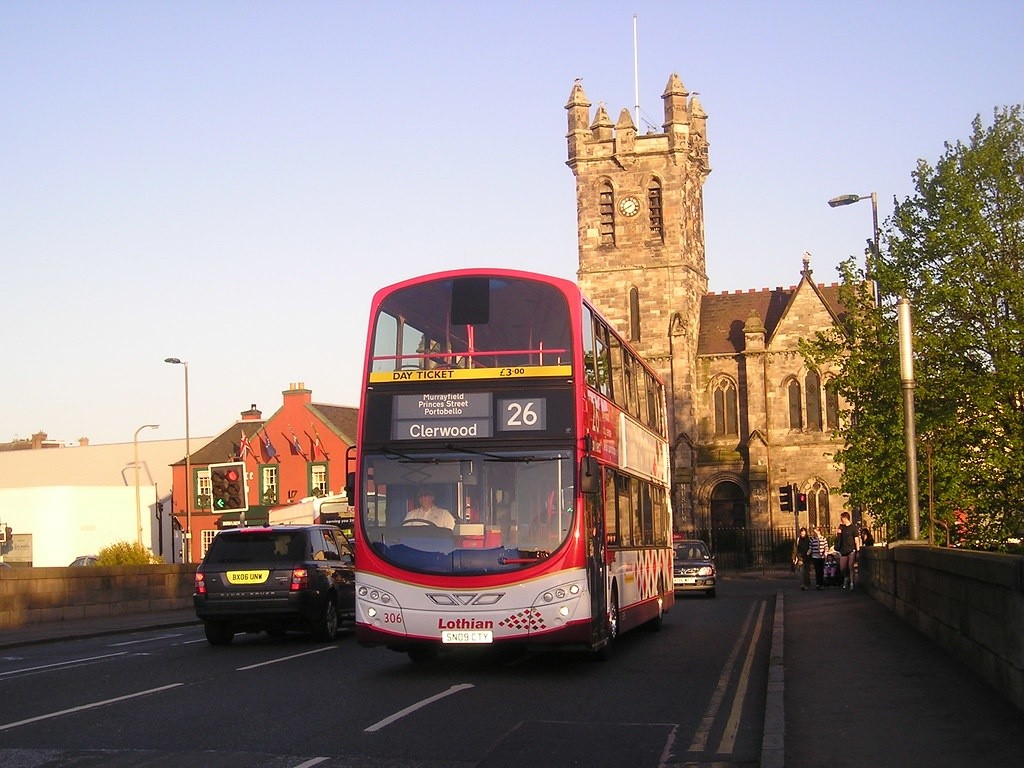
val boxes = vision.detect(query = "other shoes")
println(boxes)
[843,578,848,586]
[850,584,854,591]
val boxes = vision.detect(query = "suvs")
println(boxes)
[192,522,356,646]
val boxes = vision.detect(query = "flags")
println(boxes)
[238,426,328,456]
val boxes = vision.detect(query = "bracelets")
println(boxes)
[856,550,860,554]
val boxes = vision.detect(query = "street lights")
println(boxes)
[164,358,193,564]
[134,425,159,547]
[829,192,889,544]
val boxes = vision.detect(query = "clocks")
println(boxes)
[618,197,641,216]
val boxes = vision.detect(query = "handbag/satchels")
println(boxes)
[833,536,841,551]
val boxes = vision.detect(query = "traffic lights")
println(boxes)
[797,493,807,511]
[779,485,793,511]
[207,461,249,514]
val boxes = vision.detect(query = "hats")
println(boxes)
[417,485,434,497]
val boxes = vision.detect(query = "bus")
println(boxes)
[269,492,387,538]
[343,268,675,661]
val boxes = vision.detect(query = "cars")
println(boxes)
[673,540,717,597]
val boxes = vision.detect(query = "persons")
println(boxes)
[792,527,812,590]
[837,512,860,591]
[861,528,875,546]
[511,483,547,526]
[402,485,456,530]
[806,526,829,590]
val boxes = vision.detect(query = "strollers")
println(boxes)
[823,555,844,588]
